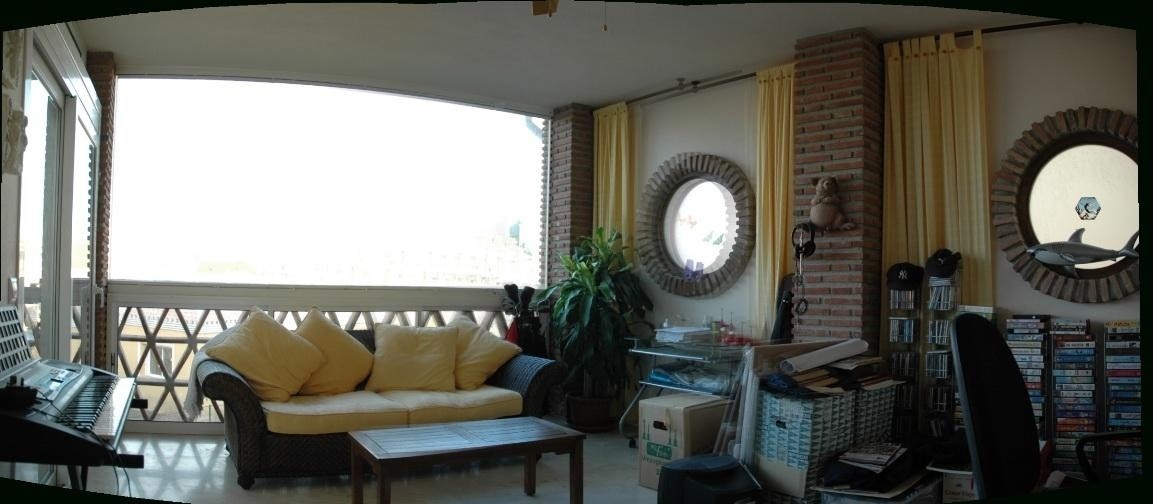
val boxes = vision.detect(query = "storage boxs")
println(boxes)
[820,476,940,504]
[759,390,855,504]
[637,392,735,491]
[943,441,1046,503]
[856,384,897,444]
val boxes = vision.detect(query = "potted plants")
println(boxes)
[533,227,654,434]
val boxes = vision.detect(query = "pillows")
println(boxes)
[446,315,523,390]
[294,306,374,396]
[207,305,325,405]
[365,324,458,392]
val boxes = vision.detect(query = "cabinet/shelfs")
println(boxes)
[888,281,920,449]
[926,277,961,453]
[619,336,747,449]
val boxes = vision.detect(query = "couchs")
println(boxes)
[197,329,573,489]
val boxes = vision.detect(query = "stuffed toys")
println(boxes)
[808,175,855,230]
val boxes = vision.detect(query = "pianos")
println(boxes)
[0,304,149,473]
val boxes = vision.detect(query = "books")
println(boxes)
[888,351,916,378]
[956,304,994,321]
[926,415,946,442]
[838,440,907,474]
[928,320,952,344]
[895,384,914,408]
[1049,318,1096,482]
[928,384,950,412]
[927,277,961,310]
[925,460,973,475]
[1103,321,1143,479]
[943,472,975,504]
[888,316,917,344]
[952,393,965,426]
[1004,319,1048,454]
[926,350,951,378]
[890,288,915,310]
[896,415,912,432]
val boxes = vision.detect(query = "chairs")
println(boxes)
[949,312,1153,503]
